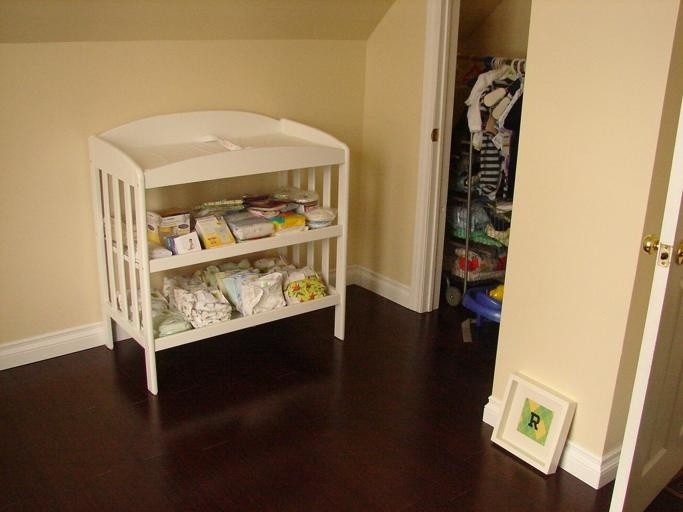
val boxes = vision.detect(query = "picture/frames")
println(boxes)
[490,373,577,475]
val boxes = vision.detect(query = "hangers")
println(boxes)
[456,54,526,89]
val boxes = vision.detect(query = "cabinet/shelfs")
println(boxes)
[441,133,511,307]
[88,110,351,395]
